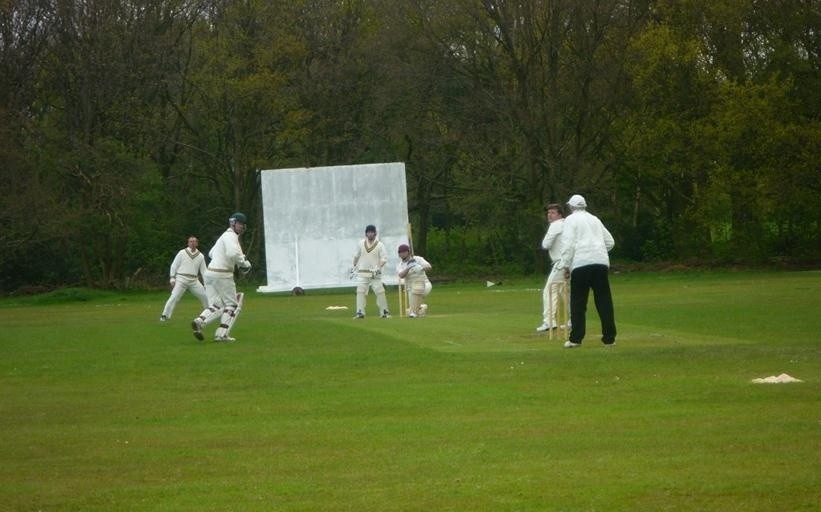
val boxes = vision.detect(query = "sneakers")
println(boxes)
[380,309,392,319]
[214,335,236,342]
[564,340,581,348]
[409,312,418,318]
[352,312,364,319]
[559,325,573,331]
[419,304,427,316]
[191,317,205,341]
[536,319,558,332]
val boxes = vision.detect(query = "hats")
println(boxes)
[565,193,588,209]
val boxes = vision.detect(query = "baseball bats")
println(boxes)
[407,224,413,261]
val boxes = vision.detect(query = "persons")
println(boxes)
[534,202,575,333]
[158,234,208,323]
[394,244,434,321]
[555,194,620,349]
[350,223,394,321]
[188,211,253,343]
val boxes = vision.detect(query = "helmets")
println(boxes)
[229,212,248,224]
[398,244,409,253]
[365,225,376,234]
